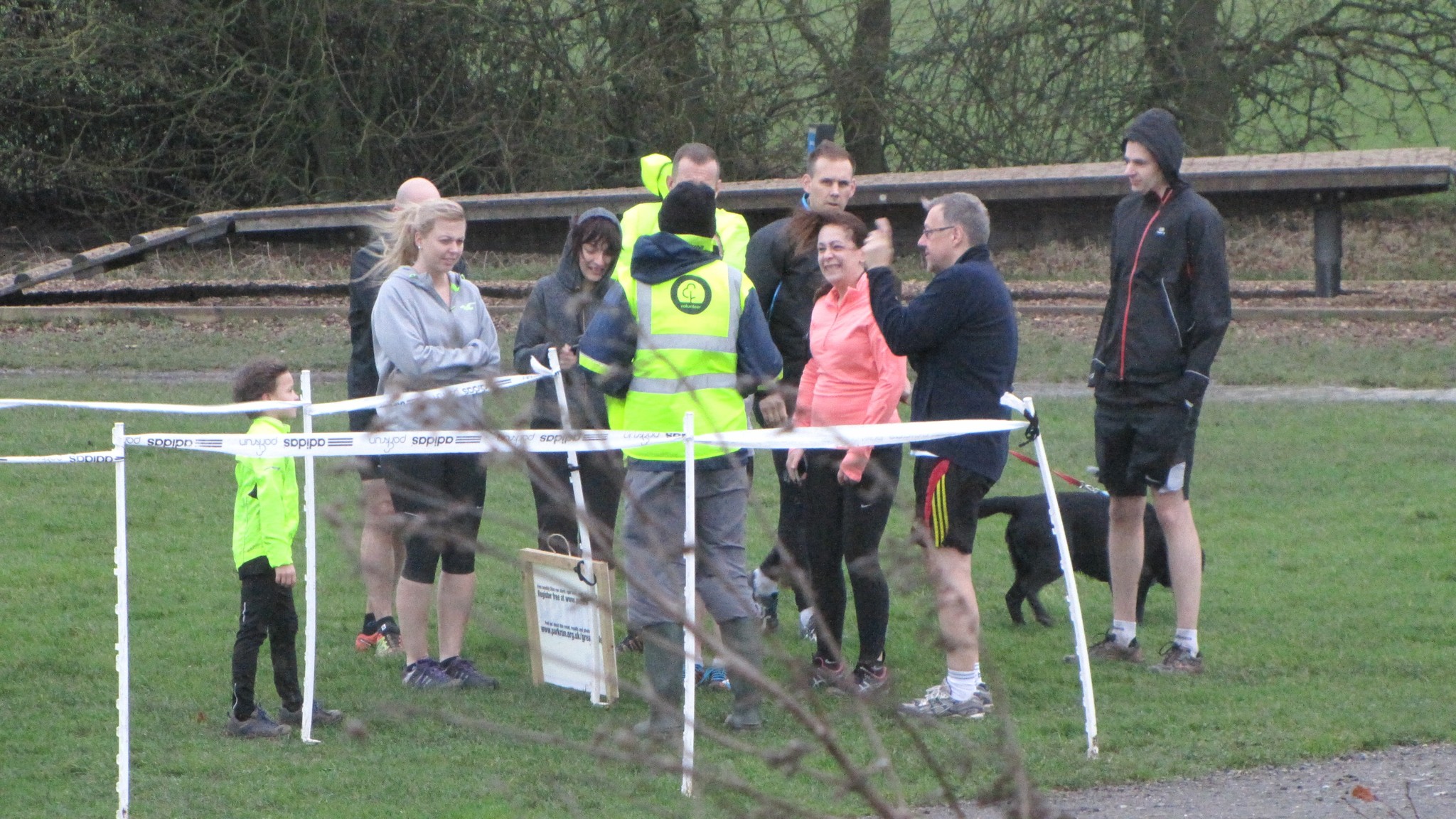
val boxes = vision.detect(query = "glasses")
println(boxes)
[923,225,966,239]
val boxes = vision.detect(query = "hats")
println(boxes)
[659,180,717,239]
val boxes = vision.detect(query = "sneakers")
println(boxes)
[1067,630,1143,667]
[375,628,402,657]
[847,661,892,696]
[792,656,848,691]
[356,632,378,651]
[1147,641,1207,677]
[800,616,818,642]
[693,663,732,693]
[279,697,341,728]
[899,687,985,720]
[749,573,780,634]
[402,656,461,691]
[441,653,498,691]
[226,703,294,739]
[920,676,993,710]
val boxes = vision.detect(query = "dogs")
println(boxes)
[976,492,1207,628]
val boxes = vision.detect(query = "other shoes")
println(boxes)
[724,703,760,731]
[635,708,685,735]
[620,637,643,654]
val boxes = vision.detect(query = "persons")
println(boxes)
[1061,109,1233,677]
[230,358,343,740]
[346,140,1022,722]
